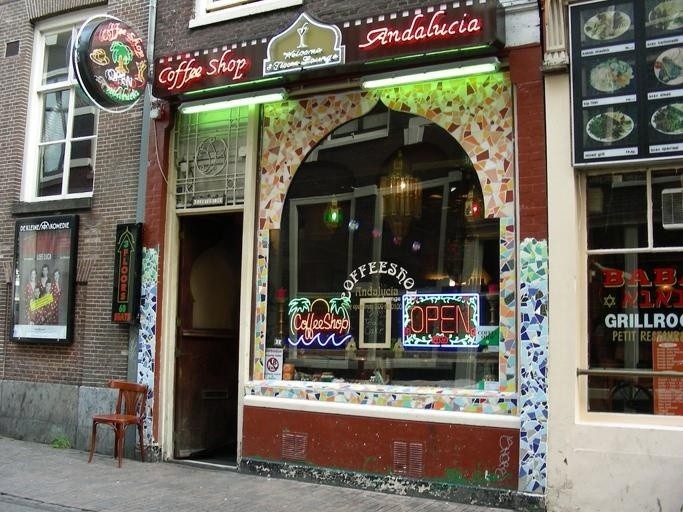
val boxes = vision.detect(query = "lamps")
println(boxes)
[375,152,423,245]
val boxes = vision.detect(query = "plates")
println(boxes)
[576,0,683,143]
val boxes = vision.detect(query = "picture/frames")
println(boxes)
[9,214,78,346]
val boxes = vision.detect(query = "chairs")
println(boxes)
[87,380,148,468]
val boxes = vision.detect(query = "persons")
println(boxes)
[22,264,63,326]
